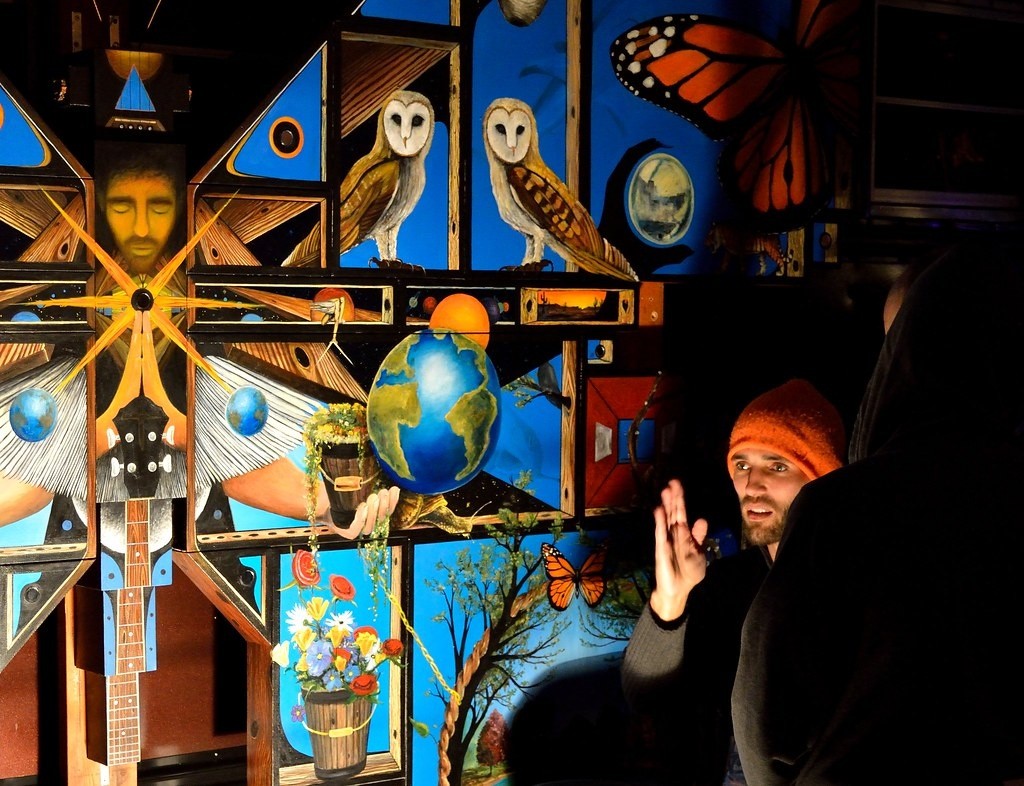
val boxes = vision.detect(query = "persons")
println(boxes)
[621,373,850,786]
[728,238,1024,786]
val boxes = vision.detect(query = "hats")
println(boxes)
[726,378,845,480]
